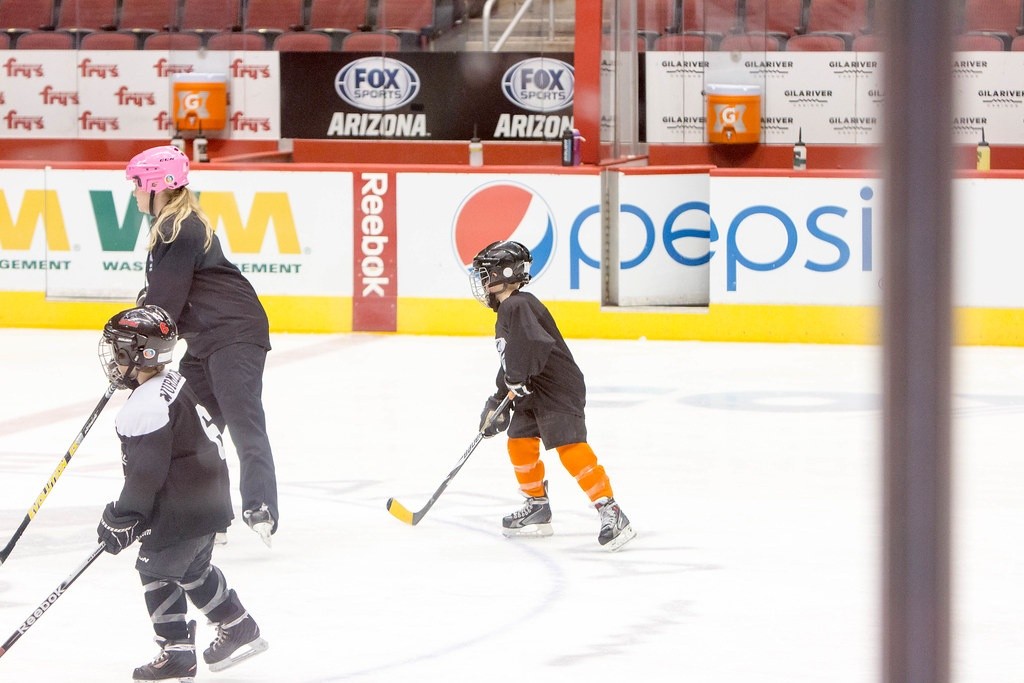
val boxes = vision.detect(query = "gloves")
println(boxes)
[135,287,148,305]
[97,502,145,555]
[504,373,534,398]
[479,395,510,436]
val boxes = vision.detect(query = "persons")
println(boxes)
[97,305,270,683]
[468,240,636,552]
[125,145,280,549]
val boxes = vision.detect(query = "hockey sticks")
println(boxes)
[0,383,117,568]
[0,541,106,659]
[385,378,531,526]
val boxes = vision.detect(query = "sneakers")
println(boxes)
[248,510,275,546]
[203,589,269,671]
[132,620,198,683]
[592,495,637,551]
[501,480,552,535]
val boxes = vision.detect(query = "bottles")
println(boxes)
[977,127,991,169]
[192,119,208,161]
[561,125,574,166]
[793,127,806,169]
[468,124,484,166]
[573,129,580,166]
[170,121,185,152]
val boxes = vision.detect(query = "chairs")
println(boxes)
[0,0,488,54]
[600,0,1024,52]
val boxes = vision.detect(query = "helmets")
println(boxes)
[127,146,189,192]
[102,305,178,389]
[473,240,532,312]
